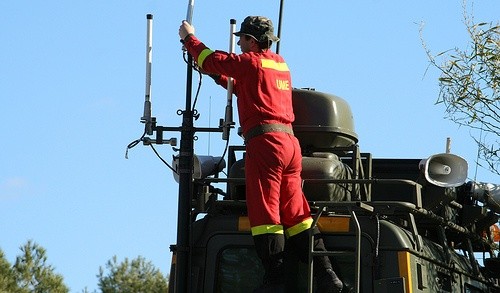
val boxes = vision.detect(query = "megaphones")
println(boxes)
[466,181,500,211]
[418,153,468,187]
[172,154,226,183]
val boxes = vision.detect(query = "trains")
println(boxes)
[187,88,499,293]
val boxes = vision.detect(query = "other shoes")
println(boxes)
[253,277,286,293]
[319,268,343,293]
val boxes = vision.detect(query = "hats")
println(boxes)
[232,15,280,44]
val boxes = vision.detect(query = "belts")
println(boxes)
[244,124,295,142]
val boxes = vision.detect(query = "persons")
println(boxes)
[179,16,343,293]
